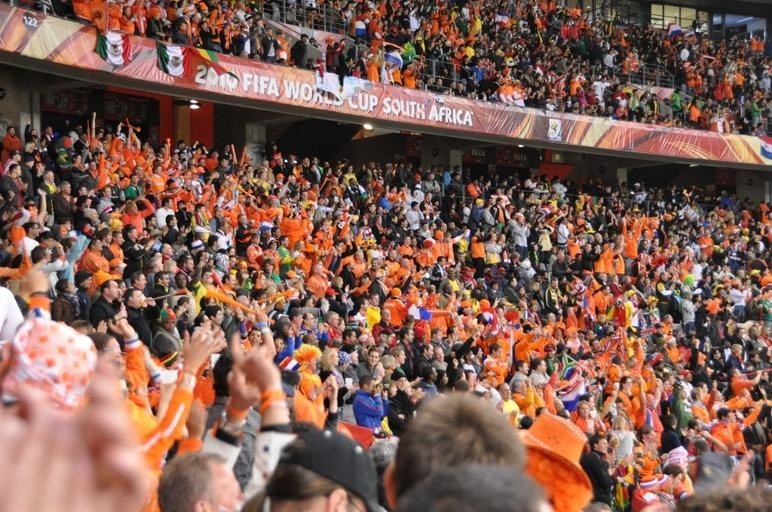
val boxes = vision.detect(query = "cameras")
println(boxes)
[374,382,389,393]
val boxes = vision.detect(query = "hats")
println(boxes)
[640,475,659,491]
[21,140,756,385]
[278,428,388,512]
[655,473,672,491]
[516,412,593,492]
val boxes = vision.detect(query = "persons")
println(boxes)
[1,0,772,511]
